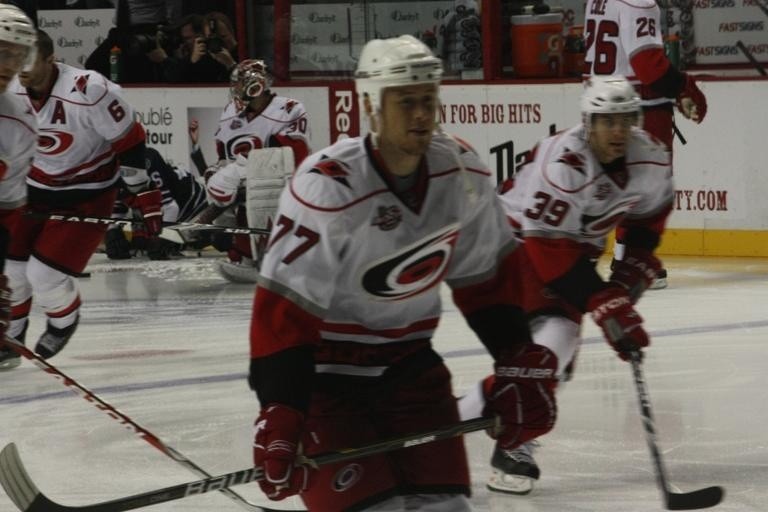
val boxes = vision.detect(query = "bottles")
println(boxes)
[663,34,679,69]
[108,44,125,82]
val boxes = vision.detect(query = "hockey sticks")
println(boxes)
[0,332,309,511]
[1,416,486,511]
[629,344,725,510]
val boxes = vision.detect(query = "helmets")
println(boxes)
[580,76,640,114]
[230,59,271,100]
[355,34,444,113]
[0,2,38,72]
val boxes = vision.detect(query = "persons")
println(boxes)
[0,28,163,371]
[66,0,237,262]
[246,35,557,512]
[1,2,39,336]
[480,75,674,480]
[581,0,708,290]
[203,56,311,282]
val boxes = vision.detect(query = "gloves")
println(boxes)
[678,75,706,122]
[254,403,312,501]
[481,344,556,449]
[589,252,661,360]
[137,190,163,235]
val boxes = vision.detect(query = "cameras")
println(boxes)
[206,36,226,53]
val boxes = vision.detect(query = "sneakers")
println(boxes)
[35,315,79,358]
[492,445,539,478]
[0,319,28,361]
